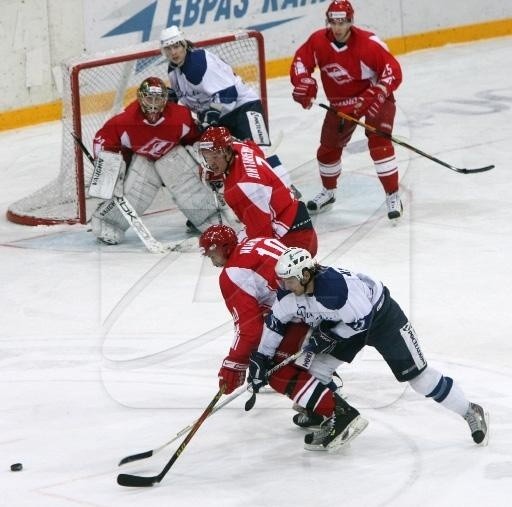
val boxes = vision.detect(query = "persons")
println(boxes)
[290,1,404,219]
[199,224,335,444]
[249,244,487,448]
[200,125,318,260]
[159,26,302,228]
[87,76,242,246]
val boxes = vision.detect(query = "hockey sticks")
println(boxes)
[63,119,200,255]
[117,383,226,486]
[118,343,311,467]
[310,98,494,173]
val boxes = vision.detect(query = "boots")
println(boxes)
[306,414,336,445]
[307,188,335,210]
[322,392,360,447]
[293,411,324,428]
[386,191,402,218]
[464,402,487,444]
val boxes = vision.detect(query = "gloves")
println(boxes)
[309,319,346,355]
[354,86,387,119]
[247,350,275,393]
[197,108,220,131]
[292,77,317,109]
[218,357,248,395]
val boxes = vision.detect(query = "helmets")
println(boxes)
[136,76,168,123]
[275,245,314,291]
[198,127,233,173]
[199,223,239,258]
[326,0,354,41]
[159,25,188,68]
